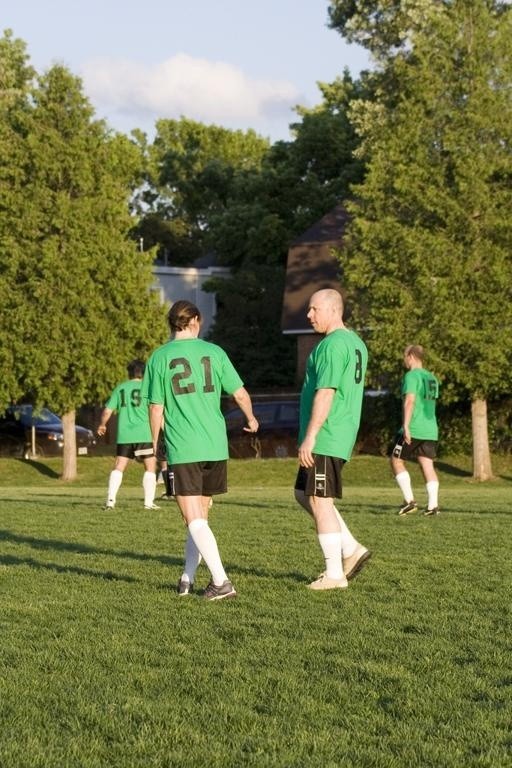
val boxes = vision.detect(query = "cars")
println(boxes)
[1,404,97,461]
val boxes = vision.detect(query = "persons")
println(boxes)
[390,345,439,517]
[140,300,260,603]
[97,359,160,510]
[293,288,371,591]
[156,459,174,501]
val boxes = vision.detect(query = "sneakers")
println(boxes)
[177,579,193,596]
[422,506,439,517]
[398,501,418,516]
[143,504,160,510]
[204,580,237,602]
[342,544,371,580]
[310,574,349,590]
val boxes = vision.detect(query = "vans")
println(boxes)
[356,391,391,456]
[224,401,300,459]
